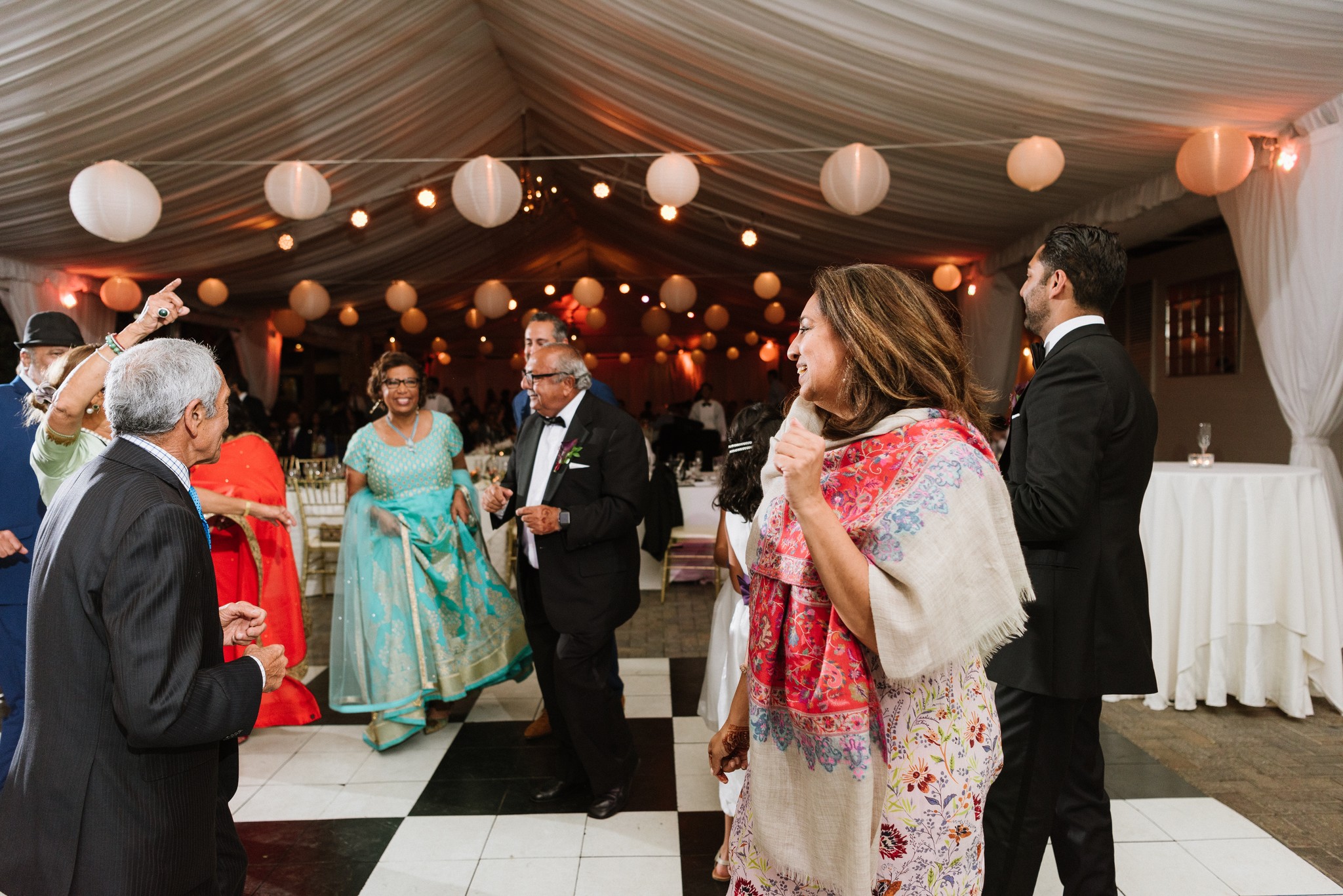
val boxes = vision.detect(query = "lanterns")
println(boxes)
[69,126,1254,368]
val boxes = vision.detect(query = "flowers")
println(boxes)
[553,438,582,472]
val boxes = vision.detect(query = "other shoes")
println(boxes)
[423,716,449,734]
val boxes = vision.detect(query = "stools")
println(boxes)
[661,525,720,603]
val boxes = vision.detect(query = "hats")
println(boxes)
[13,311,86,348]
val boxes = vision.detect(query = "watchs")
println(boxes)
[558,508,570,529]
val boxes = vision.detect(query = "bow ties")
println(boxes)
[539,415,566,428]
[1029,341,1045,371]
[702,402,711,407]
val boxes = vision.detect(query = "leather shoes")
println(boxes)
[530,778,590,802]
[524,710,551,739]
[586,759,640,818]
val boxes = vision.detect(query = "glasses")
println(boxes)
[384,377,419,389]
[521,370,564,385]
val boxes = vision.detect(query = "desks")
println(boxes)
[1101,462,1343,719]
[636,472,720,590]
[465,454,517,589]
[286,481,345,597]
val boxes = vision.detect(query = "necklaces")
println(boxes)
[387,410,418,452]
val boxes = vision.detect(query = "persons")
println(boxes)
[510,310,617,737]
[695,402,787,878]
[707,261,1034,896]
[1,312,88,781]
[0,337,289,895]
[26,279,297,531]
[181,384,319,744]
[226,370,264,438]
[265,374,515,474]
[328,351,536,754]
[981,221,1160,896]
[481,342,647,820]
[617,370,787,472]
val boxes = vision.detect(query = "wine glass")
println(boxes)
[1198,423,1212,453]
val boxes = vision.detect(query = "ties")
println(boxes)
[288,430,295,448]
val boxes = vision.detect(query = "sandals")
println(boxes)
[711,847,732,882]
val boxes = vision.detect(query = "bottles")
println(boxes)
[287,462,347,482]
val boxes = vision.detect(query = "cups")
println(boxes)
[664,451,724,476]
[469,458,510,481]
[1202,453,1215,468]
[1188,453,1201,466]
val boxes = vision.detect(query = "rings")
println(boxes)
[158,308,170,318]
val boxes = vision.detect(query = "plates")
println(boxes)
[692,480,715,487]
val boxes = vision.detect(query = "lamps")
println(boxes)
[516,115,546,216]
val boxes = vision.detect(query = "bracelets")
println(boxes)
[111,332,127,352]
[105,334,123,354]
[241,501,251,518]
[95,348,111,364]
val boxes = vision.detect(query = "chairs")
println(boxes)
[278,456,338,487]
[291,478,348,598]
